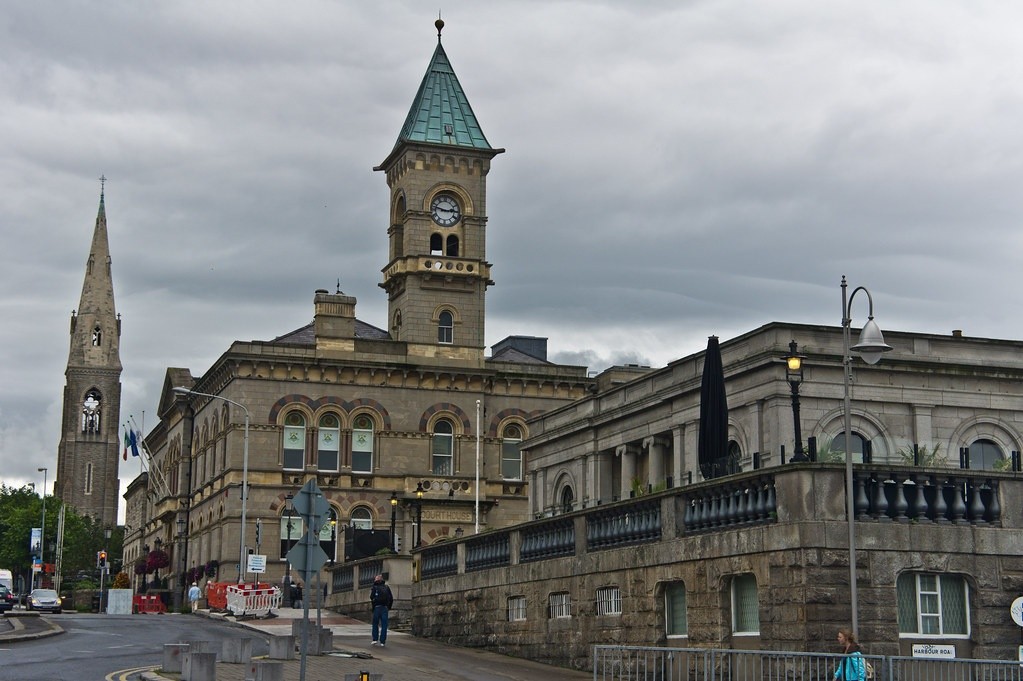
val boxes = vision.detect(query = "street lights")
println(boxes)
[48,540,56,564]
[171,386,249,586]
[839,273,894,650]
[282,491,296,608]
[142,543,150,594]
[413,481,424,549]
[103,524,113,552]
[154,536,162,588]
[785,339,810,463]
[388,490,398,555]
[113,558,118,574]
[37,467,48,589]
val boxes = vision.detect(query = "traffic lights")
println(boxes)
[255,521,262,545]
[95,553,99,569]
[98,552,106,568]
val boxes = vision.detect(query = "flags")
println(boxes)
[130,427,139,456]
[122,430,131,461]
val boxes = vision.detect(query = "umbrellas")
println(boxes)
[698,334,729,479]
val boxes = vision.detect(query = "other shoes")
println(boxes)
[371,641,378,645]
[380,644,385,647]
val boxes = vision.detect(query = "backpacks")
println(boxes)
[372,584,391,607]
[849,650,874,680]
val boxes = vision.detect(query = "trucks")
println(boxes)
[0,569,13,594]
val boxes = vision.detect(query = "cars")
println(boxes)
[26,589,66,614]
[62,569,99,583]
[0,584,14,613]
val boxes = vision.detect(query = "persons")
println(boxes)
[292,583,304,609]
[189,582,202,614]
[369,575,393,647]
[204,580,211,609]
[290,581,297,607]
[833,629,865,681]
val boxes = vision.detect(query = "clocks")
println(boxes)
[430,195,462,227]
